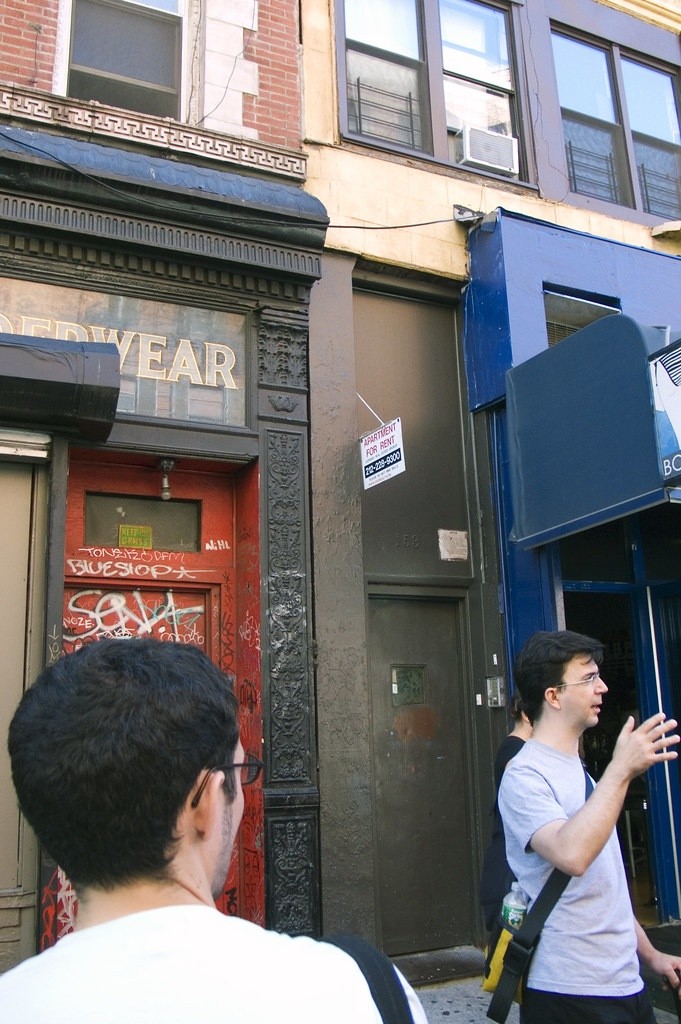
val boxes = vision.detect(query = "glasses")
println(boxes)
[191,751,265,807]
[553,673,600,687]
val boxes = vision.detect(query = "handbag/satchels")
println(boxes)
[479,910,542,1023]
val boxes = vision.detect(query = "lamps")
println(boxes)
[160,471,172,500]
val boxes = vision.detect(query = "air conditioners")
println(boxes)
[453,124,519,178]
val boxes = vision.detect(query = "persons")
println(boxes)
[0,637,429,1024]
[478,630,681,1024]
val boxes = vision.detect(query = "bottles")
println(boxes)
[502,882,528,931]
